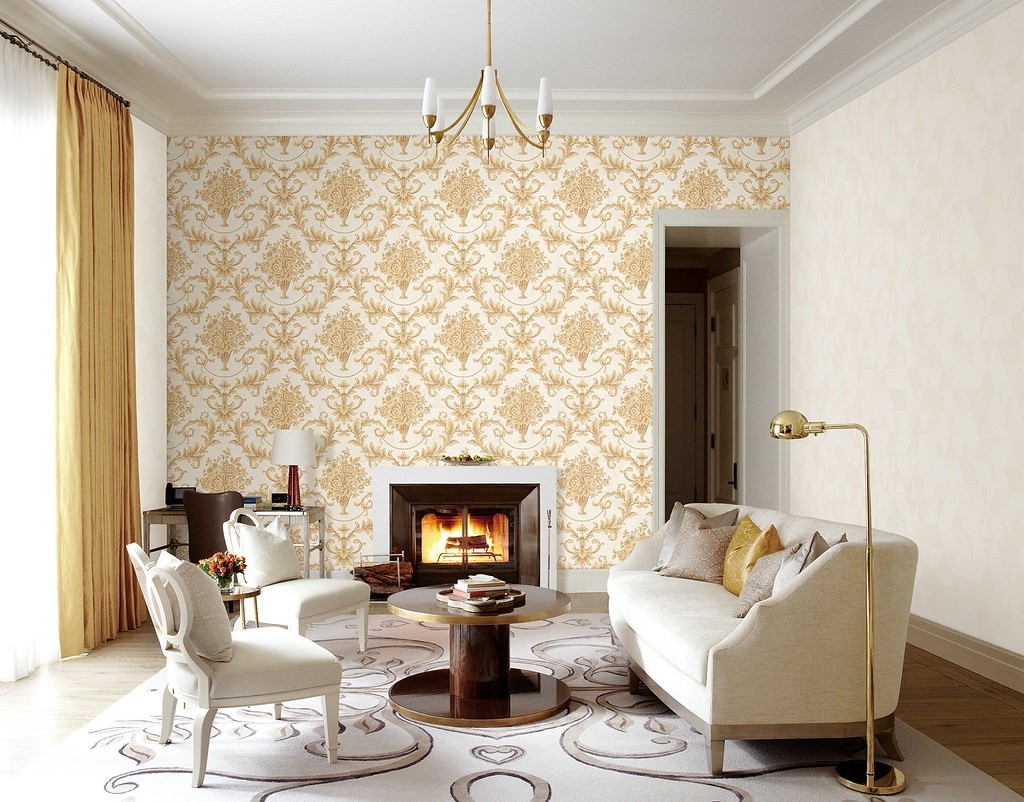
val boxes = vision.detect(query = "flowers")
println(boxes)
[196,550,247,578]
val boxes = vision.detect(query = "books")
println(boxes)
[243,497,264,510]
[452,574,509,599]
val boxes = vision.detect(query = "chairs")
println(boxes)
[232,508,371,655]
[125,542,344,790]
[183,490,244,564]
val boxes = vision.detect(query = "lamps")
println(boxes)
[421,0,554,156]
[272,426,318,510]
[770,412,909,795]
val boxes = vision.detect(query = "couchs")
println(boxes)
[608,502,918,779]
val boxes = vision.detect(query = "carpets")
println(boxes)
[0,611,1024,802]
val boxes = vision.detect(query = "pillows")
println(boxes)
[153,549,235,660]
[722,514,784,596]
[221,515,301,587]
[659,510,740,585]
[771,531,834,598]
[651,502,739,571]
[735,541,802,618]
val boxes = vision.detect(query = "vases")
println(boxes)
[216,575,234,593]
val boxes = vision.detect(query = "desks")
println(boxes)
[220,586,260,629]
[385,582,572,728]
[143,508,189,562]
[254,506,327,579]
